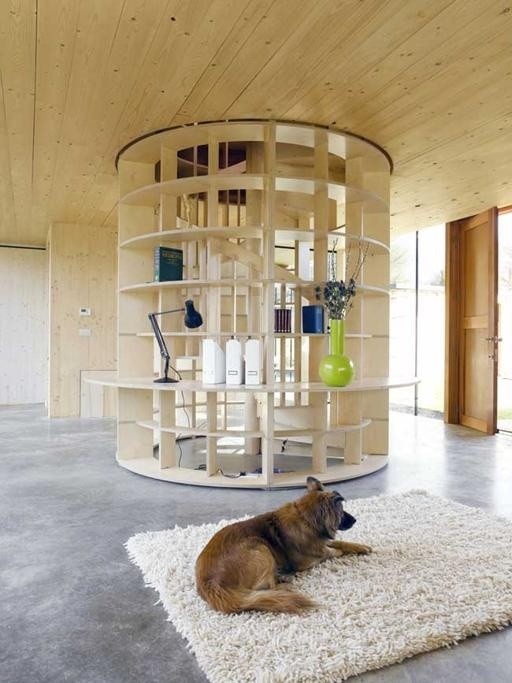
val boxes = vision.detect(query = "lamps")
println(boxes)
[149,297,203,383]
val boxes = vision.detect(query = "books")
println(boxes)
[154,246,184,282]
[302,305,324,334]
[273,308,292,333]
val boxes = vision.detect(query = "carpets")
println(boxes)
[124,489,511,683]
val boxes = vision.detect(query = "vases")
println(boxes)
[318,320,356,386]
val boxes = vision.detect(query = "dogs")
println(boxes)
[195,476,370,619]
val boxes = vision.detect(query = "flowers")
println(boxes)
[315,279,360,321]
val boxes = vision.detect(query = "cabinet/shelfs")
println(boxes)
[116,117,393,492]
